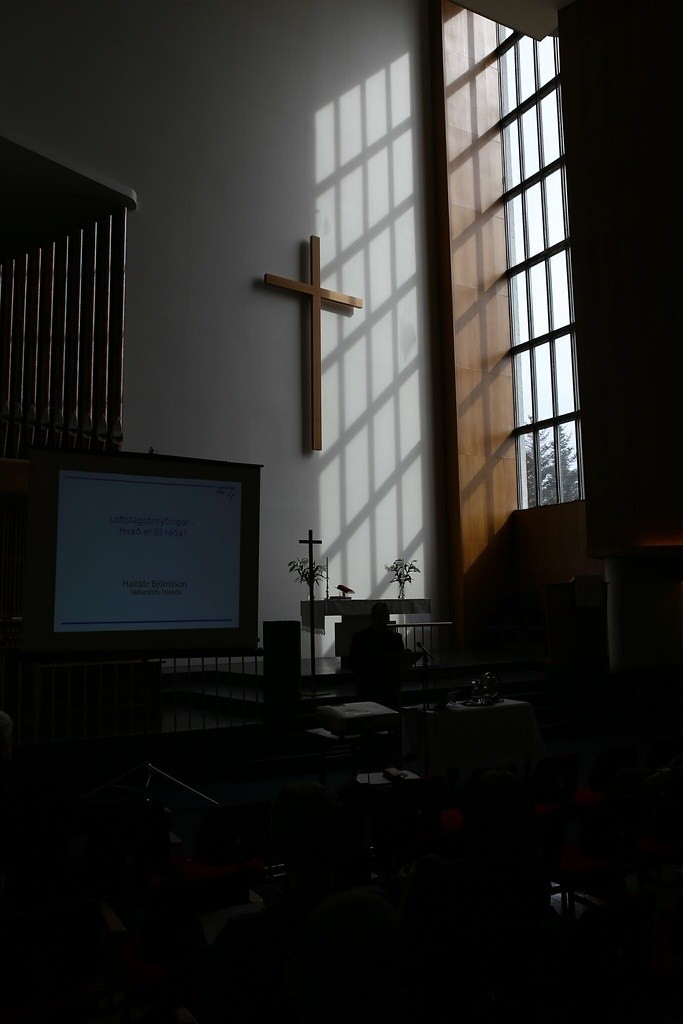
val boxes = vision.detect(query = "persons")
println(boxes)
[0,710,403,1024]
[340,601,411,702]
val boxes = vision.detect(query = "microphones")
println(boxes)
[417,642,432,658]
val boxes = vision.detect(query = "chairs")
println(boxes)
[0,753,682,1024]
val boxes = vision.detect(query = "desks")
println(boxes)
[401,697,547,801]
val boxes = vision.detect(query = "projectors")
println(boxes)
[316,701,402,735]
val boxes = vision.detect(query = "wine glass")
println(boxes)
[470,681,481,702]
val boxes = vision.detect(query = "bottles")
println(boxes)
[483,673,493,703]
